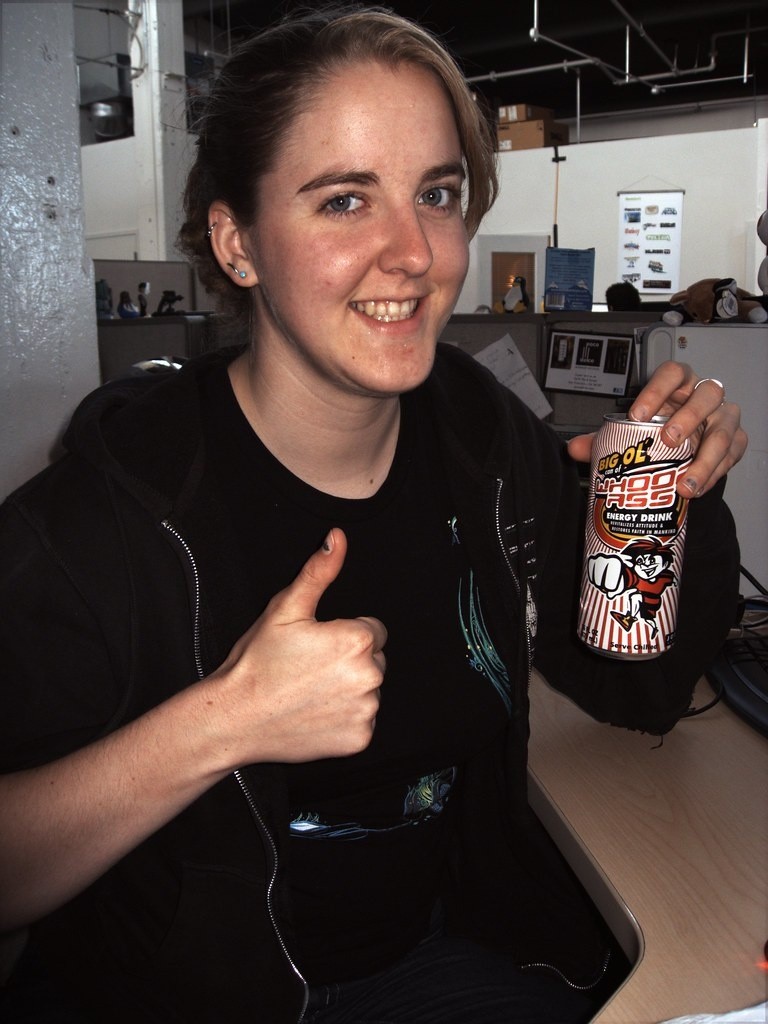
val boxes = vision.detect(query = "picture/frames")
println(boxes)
[540,327,636,400]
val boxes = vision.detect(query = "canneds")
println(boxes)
[575,411,693,661]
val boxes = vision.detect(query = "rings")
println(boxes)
[693,377,725,411]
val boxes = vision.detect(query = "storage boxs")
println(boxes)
[497,119,569,151]
[498,104,555,124]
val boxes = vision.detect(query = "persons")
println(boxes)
[2,4,751,1024]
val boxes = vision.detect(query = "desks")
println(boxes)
[517,666,768,1024]
